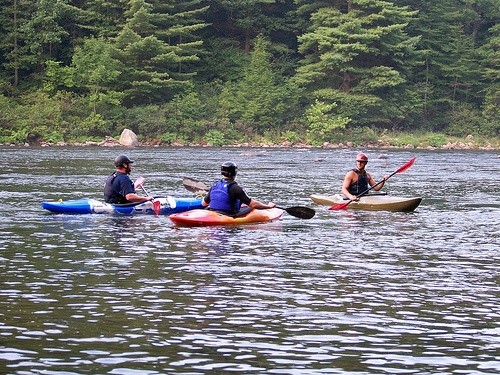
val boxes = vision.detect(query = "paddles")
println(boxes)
[181,176,316,220]
[134,177,161,215]
[327,157,417,211]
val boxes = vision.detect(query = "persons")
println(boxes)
[104,156,154,204]
[342,153,389,201]
[201,161,276,213]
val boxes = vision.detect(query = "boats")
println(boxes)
[168,208,285,226]
[310,190,422,214]
[42,196,207,214]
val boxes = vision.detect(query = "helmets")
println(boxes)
[115,156,134,167]
[221,161,238,173]
[356,153,368,162]
